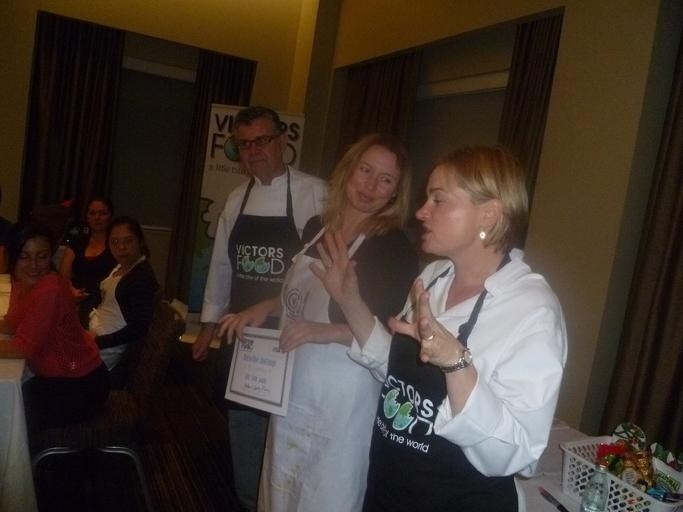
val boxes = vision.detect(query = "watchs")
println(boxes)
[435,347,474,373]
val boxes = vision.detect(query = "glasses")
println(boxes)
[233,131,283,151]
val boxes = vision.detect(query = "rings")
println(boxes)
[326,263,334,268]
[423,331,436,341]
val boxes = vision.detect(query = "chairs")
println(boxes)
[34,297,184,509]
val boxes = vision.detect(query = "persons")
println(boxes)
[188,107,333,512]
[0,195,160,435]
[309,148,567,511]
[219,128,417,511]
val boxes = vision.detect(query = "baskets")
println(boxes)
[560,436,682,512]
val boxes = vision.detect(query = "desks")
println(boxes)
[0,273,41,510]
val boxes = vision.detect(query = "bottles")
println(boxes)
[579,462,611,512]
